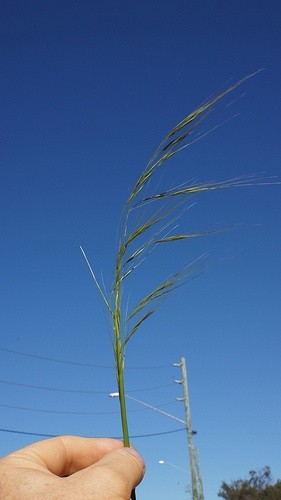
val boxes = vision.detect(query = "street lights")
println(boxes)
[109,358,199,500]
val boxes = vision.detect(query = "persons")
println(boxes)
[1,430,145,500]
